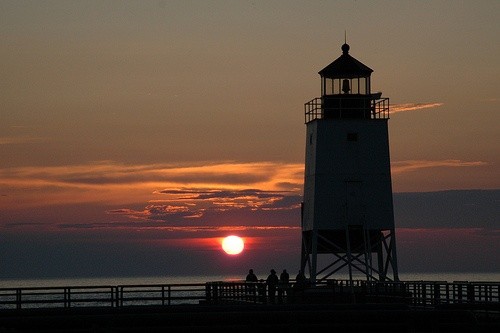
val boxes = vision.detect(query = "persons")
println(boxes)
[246,267,307,299]
